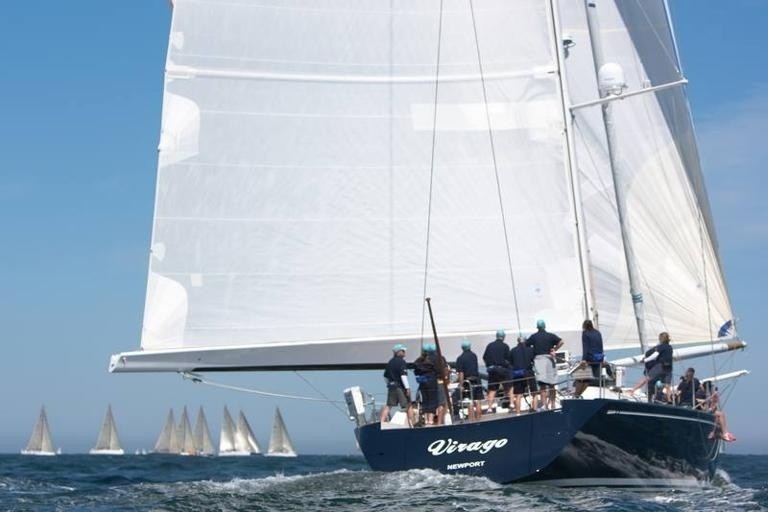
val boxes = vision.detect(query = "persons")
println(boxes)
[427,343,449,423]
[581,319,616,387]
[452,340,485,420]
[483,331,516,414]
[510,336,538,416]
[414,344,441,427]
[379,344,419,428]
[676,367,736,442]
[410,400,421,428]
[524,320,564,411]
[624,332,673,404]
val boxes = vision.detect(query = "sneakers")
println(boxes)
[483,404,547,416]
[720,432,736,442]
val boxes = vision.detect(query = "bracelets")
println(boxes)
[444,376,449,379]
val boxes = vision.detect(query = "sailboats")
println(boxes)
[88,404,124,456]
[150,404,299,461]
[104,2,754,481]
[20,401,58,457]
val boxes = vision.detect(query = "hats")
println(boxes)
[537,319,546,330]
[462,341,471,351]
[495,329,506,338]
[422,342,437,353]
[392,344,408,354]
